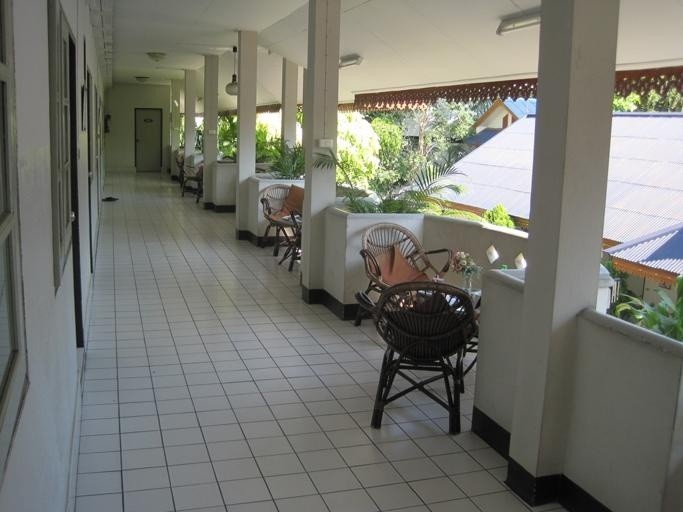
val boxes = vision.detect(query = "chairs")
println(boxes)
[353,221,454,327]
[260,183,300,256]
[279,210,302,273]
[175,151,234,203]
[354,282,474,435]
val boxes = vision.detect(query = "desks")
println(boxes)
[276,216,304,259]
[400,292,478,382]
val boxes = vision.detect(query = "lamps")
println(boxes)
[225,46,239,95]
[144,52,167,65]
[338,54,364,68]
[495,5,540,36]
[135,76,151,84]
